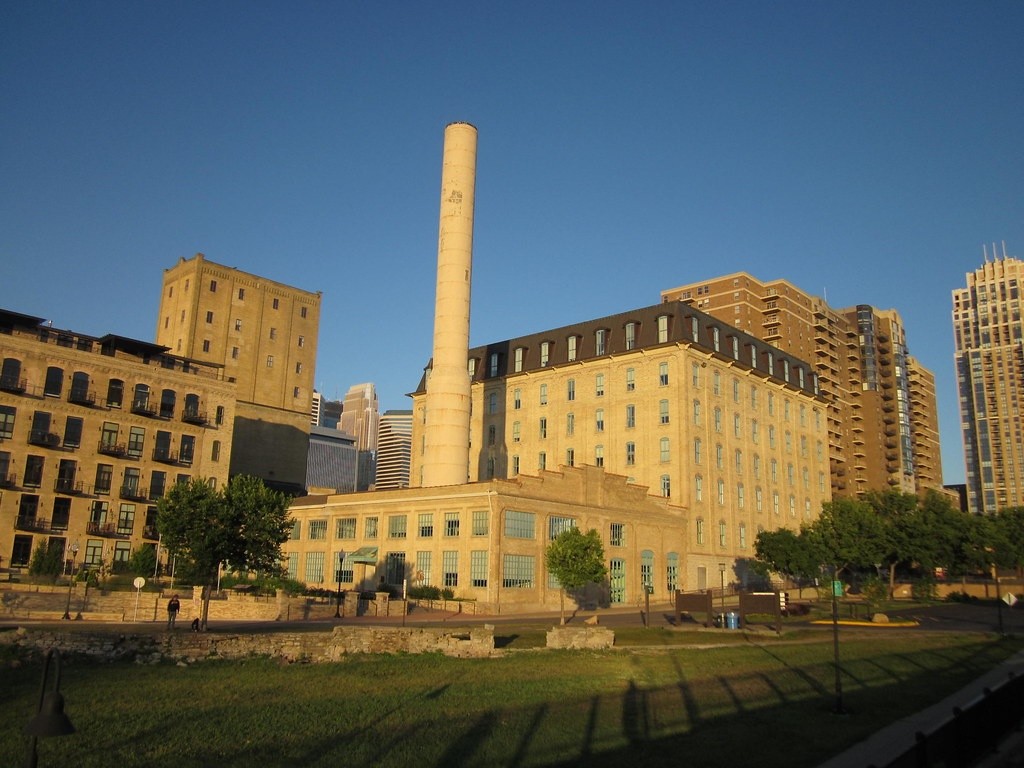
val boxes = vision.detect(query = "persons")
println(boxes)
[164,594,181,630]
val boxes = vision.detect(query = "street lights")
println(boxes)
[718,563,725,611]
[25,647,76,768]
[62,545,80,620]
[334,549,345,619]
